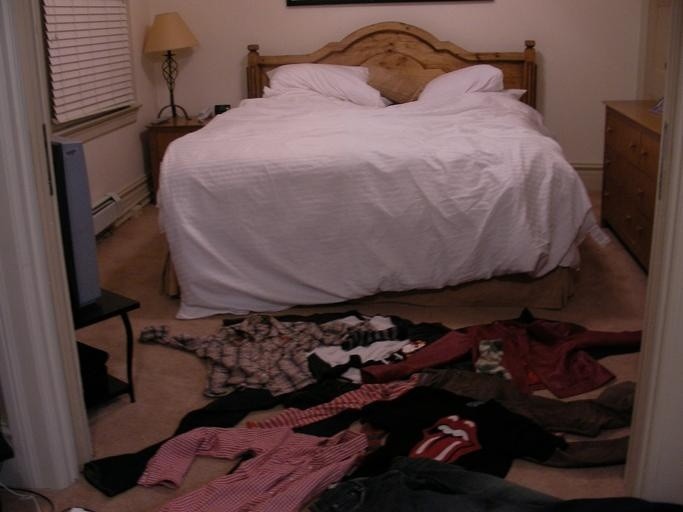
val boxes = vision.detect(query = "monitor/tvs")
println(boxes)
[51,136,102,308]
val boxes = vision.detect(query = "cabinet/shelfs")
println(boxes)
[600,99,663,282]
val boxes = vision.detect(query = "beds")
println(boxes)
[161,22,569,311]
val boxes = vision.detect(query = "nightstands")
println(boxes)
[140,116,210,202]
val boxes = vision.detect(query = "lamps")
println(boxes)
[142,9,201,126]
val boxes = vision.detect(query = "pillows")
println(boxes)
[417,64,529,113]
[258,61,388,108]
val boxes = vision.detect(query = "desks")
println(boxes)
[69,290,140,409]
